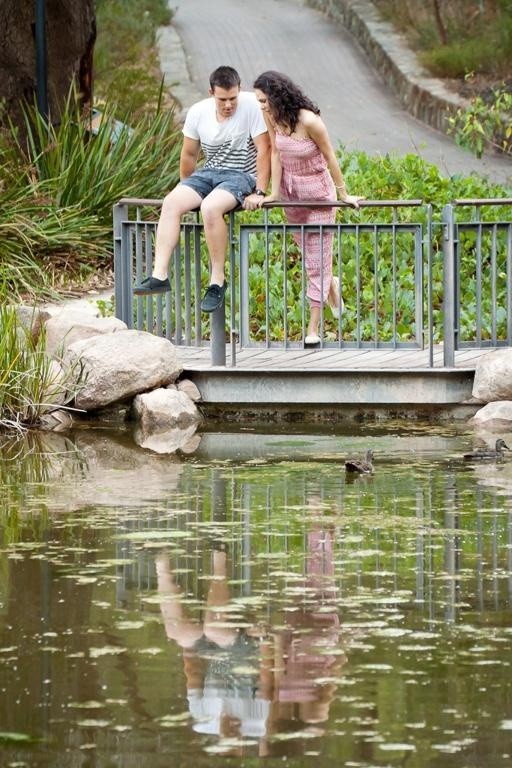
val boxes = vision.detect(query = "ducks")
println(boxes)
[345,448,375,476]
[464,438,509,460]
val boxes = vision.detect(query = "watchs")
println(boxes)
[253,189,265,197]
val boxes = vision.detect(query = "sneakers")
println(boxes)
[305,335,320,344]
[133,277,171,296]
[330,277,344,317]
[201,279,227,312]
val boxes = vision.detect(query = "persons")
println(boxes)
[142,515,273,759]
[258,471,353,755]
[131,63,273,312]
[253,70,366,348]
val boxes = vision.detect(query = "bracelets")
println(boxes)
[335,181,346,188]
